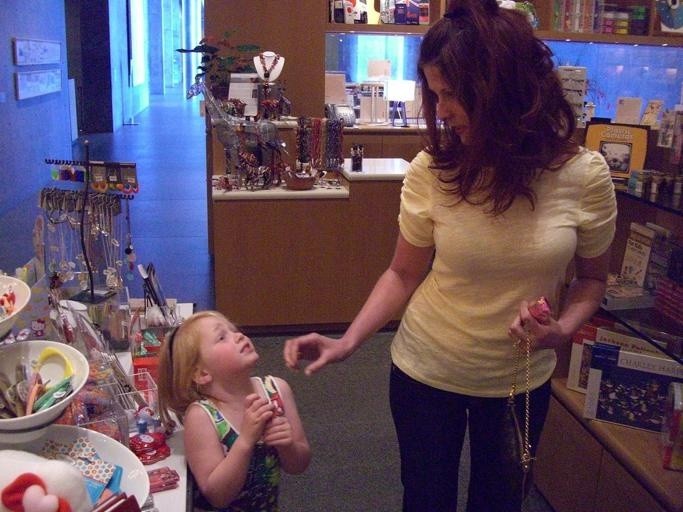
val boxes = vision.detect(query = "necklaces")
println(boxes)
[259,53,280,88]
[505,317,535,475]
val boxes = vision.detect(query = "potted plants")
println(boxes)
[175,26,260,98]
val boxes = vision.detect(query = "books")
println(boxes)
[563,220,682,436]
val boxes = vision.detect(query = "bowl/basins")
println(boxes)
[0,273,31,339]
[0,424,151,512]
[0,339,90,443]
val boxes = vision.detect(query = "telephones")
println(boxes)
[326,103,356,127]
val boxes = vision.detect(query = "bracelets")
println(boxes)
[0,343,77,421]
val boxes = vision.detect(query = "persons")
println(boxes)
[155,309,312,510]
[279,0,619,509]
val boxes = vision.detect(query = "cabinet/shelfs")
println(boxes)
[204,0,683,512]
